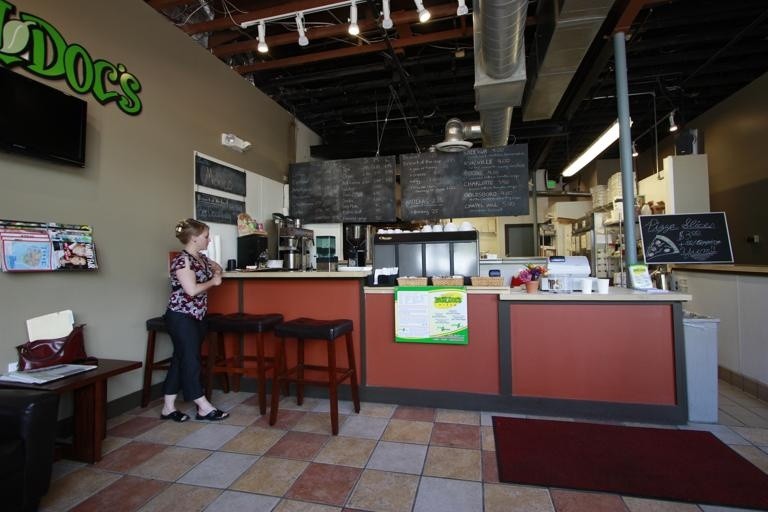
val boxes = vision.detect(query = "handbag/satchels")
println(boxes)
[16,321,90,373]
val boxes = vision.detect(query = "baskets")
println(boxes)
[396,277,428,287]
[470,277,505,287]
[431,277,464,286]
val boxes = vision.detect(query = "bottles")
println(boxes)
[228,259,236,270]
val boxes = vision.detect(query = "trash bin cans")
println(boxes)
[683,309,721,425]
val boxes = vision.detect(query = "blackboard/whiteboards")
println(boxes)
[638,211,734,265]
[288,154,395,223]
[399,143,529,220]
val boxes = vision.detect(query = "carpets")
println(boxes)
[489,414,768,511]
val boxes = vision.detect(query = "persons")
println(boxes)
[160,218,229,423]
[52,252,91,268]
[64,242,93,259]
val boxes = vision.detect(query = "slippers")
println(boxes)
[197,409,229,421]
[160,410,191,422]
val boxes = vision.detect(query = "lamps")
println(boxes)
[236,1,473,56]
[555,111,634,180]
[221,132,253,155]
[629,107,679,158]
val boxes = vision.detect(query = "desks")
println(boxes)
[0,353,142,465]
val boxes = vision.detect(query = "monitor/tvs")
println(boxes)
[0,66,87,167]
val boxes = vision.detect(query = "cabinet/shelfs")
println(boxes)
[570,169,667,293]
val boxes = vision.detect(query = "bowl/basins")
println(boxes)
[246,265,258,270]
[377,222,474,235]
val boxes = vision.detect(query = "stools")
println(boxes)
[203,311,292,416]
[269,317,362,437]
[139,311,229,412]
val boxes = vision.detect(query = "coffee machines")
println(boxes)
[346,225,376,268]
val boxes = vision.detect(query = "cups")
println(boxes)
[580,277,610,295]
[202,235,222,266]
